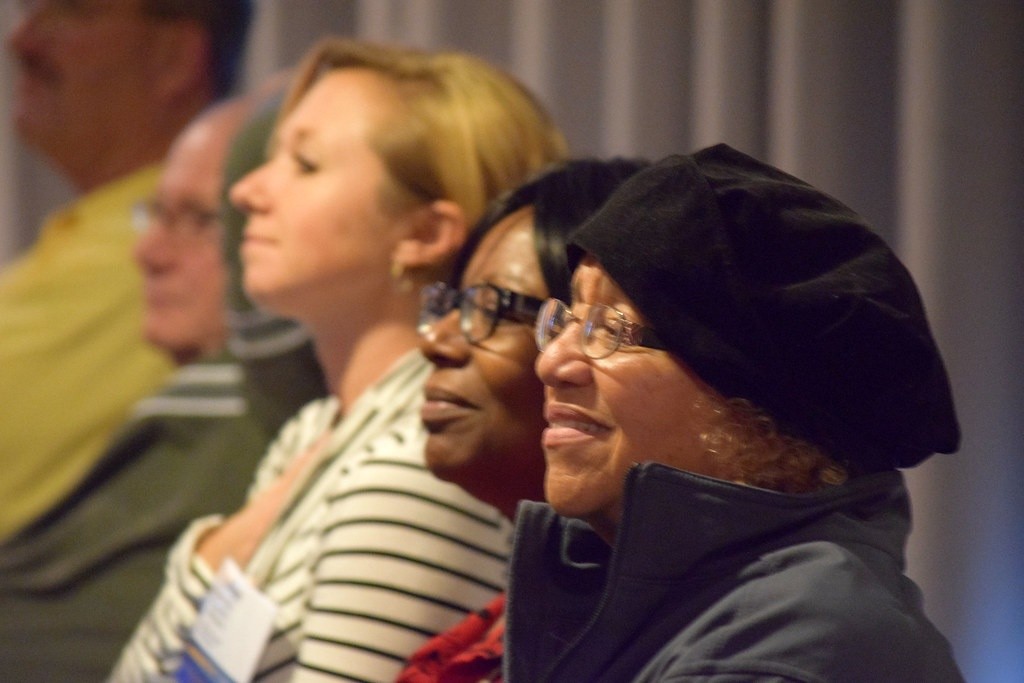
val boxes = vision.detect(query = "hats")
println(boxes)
[563,142,962,481]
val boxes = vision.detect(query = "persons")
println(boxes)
[507,135,970,683]
[2,70,329,683]
[2,0,255,532]
[402,154,653,683]
[101,36,524,680]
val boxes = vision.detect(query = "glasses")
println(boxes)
[533,296,671,361]
[414,278,546,346]
